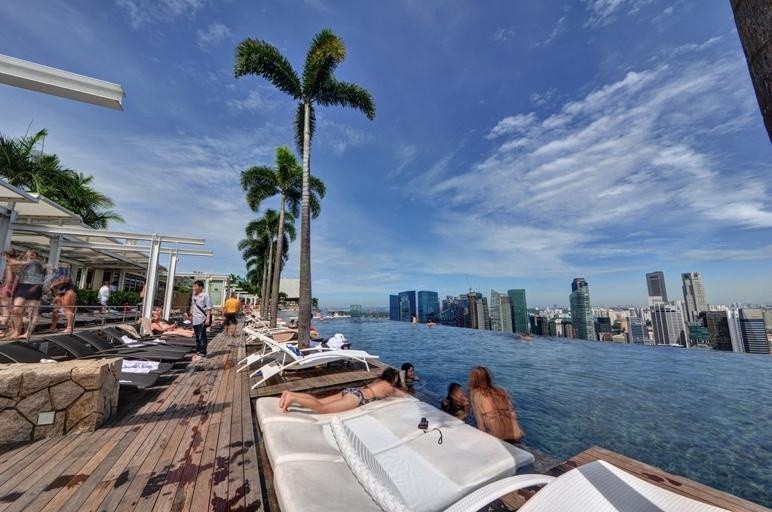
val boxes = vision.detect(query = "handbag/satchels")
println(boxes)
[204,315,212,327]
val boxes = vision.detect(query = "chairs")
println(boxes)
[0,313,195,397]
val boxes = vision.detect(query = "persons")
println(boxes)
[97,281,111,310]
[412,314,417,328]
[192,280,213,356]
[280,367,400,414]
[439,383,470,419]
[223,293,241,337]
[468,366,524,444]
[288,319,295,327]
[151,309,194,339]
[1,249,76,338]
[402,362,419,382]
